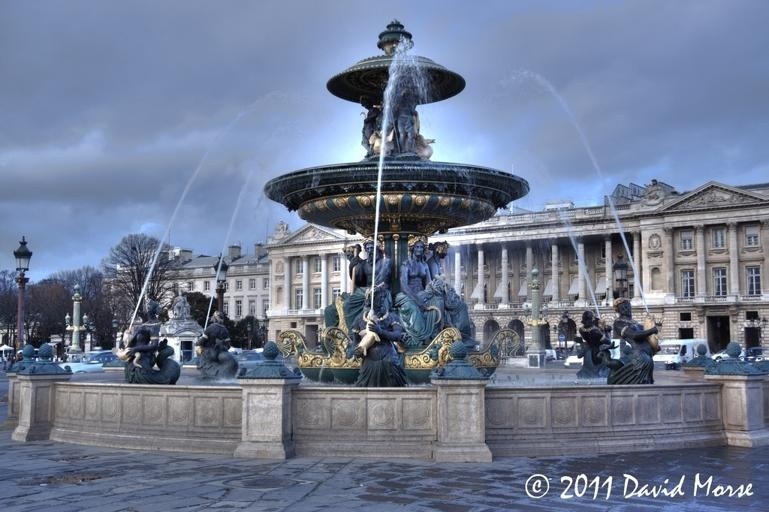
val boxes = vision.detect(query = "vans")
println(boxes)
[652,338,711,369]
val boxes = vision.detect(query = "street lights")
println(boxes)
[12,236,33,350]
[612,254,629,298]
[213,252,229,311]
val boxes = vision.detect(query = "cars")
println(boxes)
[711,346,769,362]
[58,350,120,374]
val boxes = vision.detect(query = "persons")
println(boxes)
[360,88,436,159]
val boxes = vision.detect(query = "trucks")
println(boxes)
[564,338,632,369]
